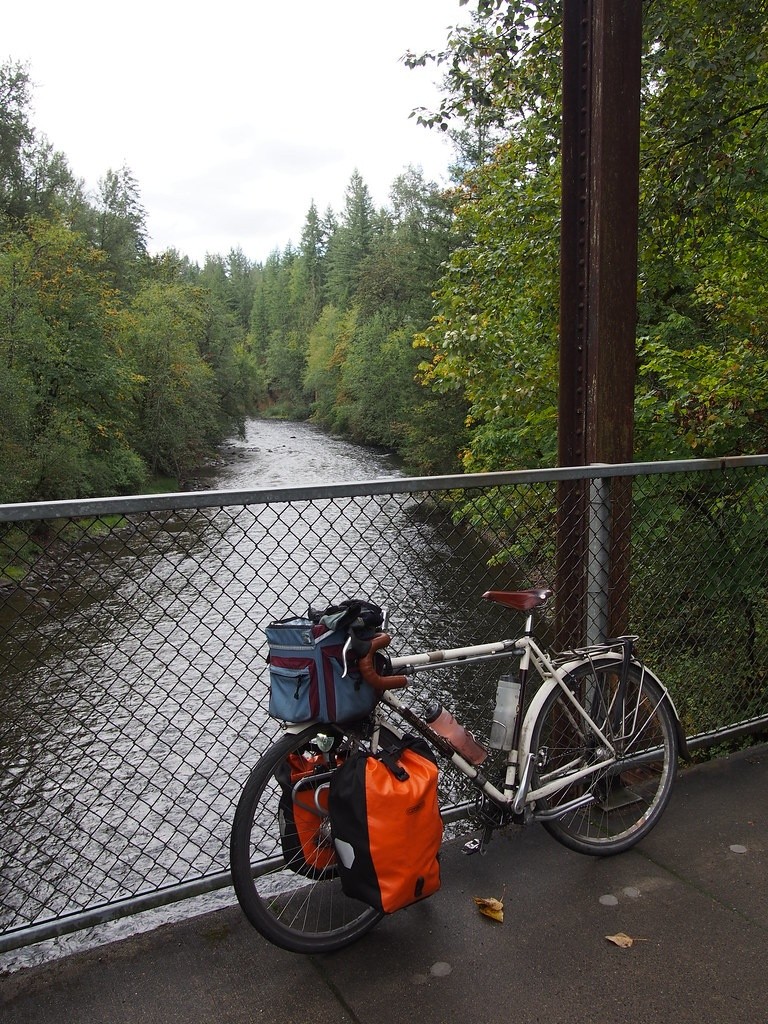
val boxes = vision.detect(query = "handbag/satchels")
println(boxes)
[326,732,443,913]
[265,616,376,725]
[273,752,345,881]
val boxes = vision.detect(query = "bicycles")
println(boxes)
[229,586,688,954]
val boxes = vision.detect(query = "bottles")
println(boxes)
[489,671,521,751]
[422,702,488,766]
